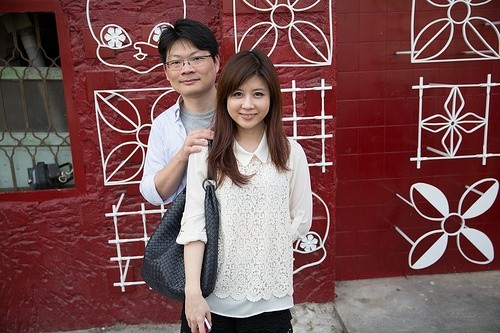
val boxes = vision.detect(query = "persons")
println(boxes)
[139,19,222,206]
[177,50,313,333]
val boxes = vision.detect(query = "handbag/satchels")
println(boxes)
[140,139,221,302]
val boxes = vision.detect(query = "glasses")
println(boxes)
[164,54,214,71]
